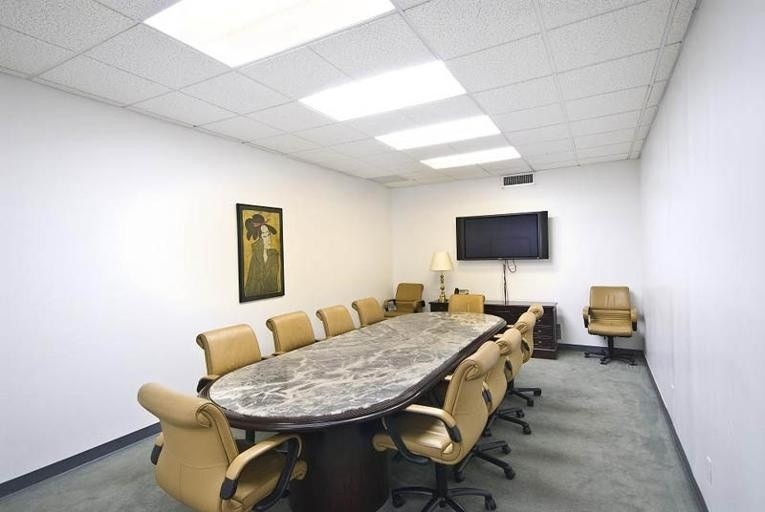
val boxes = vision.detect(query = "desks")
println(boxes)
[206,306,509,511]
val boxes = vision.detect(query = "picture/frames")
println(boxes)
[233,202,286,303]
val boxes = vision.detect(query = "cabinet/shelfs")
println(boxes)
[428,297,561,360]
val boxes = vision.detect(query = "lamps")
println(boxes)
[428,249,454,303]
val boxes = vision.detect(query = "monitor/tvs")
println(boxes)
[456,210,548,260]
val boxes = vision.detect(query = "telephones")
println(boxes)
[454,288,469,295]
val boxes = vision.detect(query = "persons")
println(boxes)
[244,215,278,297]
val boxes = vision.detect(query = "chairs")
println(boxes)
[134,381,311,512]
[446,293,486,315]
[582,284,640,367]
[191,294,387,453]
[365,301,549,511]
[381,282,426,318]
